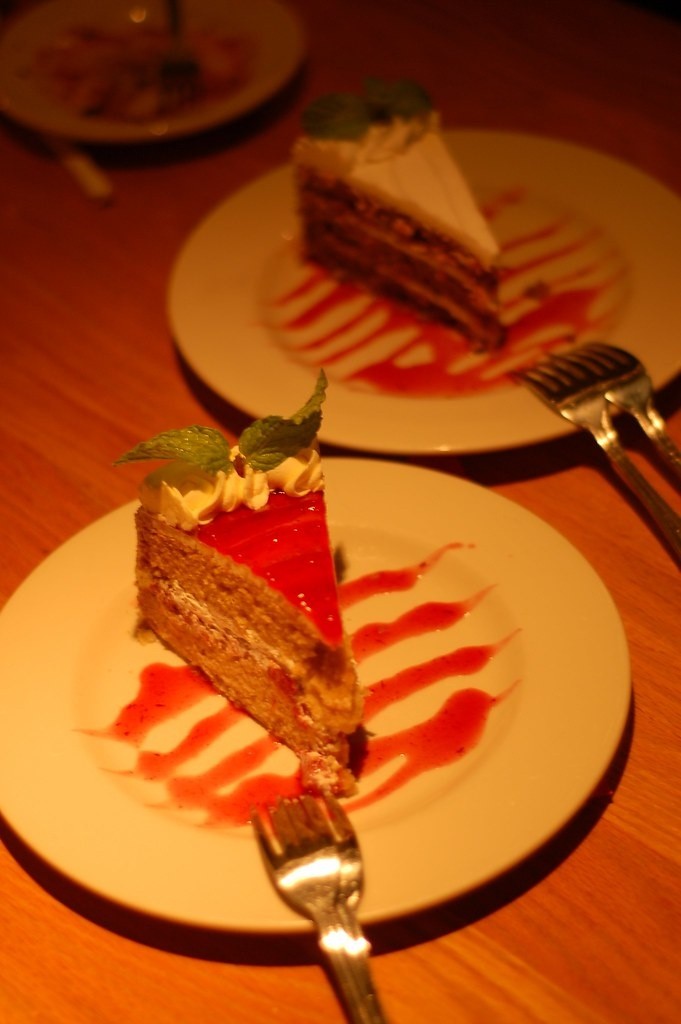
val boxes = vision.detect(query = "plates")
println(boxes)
[0,459,633,936]
[171,125,680,457]
[0,0,308,142]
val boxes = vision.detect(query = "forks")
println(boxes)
[569,341,680,495]
[254,782,404,1024]
[513,353,681,565]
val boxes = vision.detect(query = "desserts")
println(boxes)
[291,71,510,351]
[111,367,363,801]
